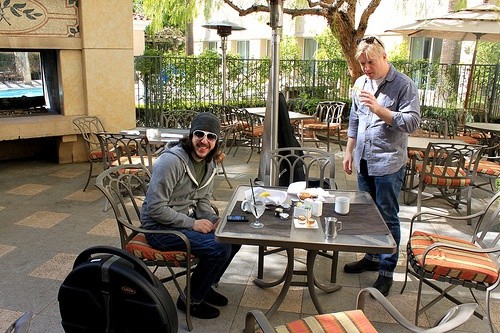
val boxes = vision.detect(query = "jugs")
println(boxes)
[325,217,342,239]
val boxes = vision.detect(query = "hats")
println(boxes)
[190,112,220,140]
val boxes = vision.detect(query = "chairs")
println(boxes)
[95,162,219,331]
[245,287,477,333]
[398,192,500,333]
[298,101,346,152]
[72,102,264,213]
[401,108,500,225]
[258,147,339,283]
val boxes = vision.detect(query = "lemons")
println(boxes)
[260,192,271,197]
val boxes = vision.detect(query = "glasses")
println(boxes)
[356,36,384,48]
[191,130,218,142]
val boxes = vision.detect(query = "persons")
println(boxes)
[142,113,242,319]
[344,37,421,298]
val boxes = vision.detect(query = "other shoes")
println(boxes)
[185,283,228,306]
[344,258,380,274]
[176,294,220,319]
[369,274,393,299]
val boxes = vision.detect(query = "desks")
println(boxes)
[407,136,479,155]
[101,126,190,156]
[214,183,397,332]
[232,106,317,152]
[465,122,500,162]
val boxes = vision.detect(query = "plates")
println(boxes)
[294,219,319,228]
[297,194,326,202]
[252,188,288,205]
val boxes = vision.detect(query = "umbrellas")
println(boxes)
[384,0,500,124]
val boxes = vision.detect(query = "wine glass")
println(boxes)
[249,200,266,228]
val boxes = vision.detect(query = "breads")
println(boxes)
[299,192,312,200]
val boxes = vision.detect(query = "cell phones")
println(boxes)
[227,215,249,222]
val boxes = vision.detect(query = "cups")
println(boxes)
[312,200,322,216]
[275,205,284,217]
[241,195,255,213]
[335,196,350,215]
